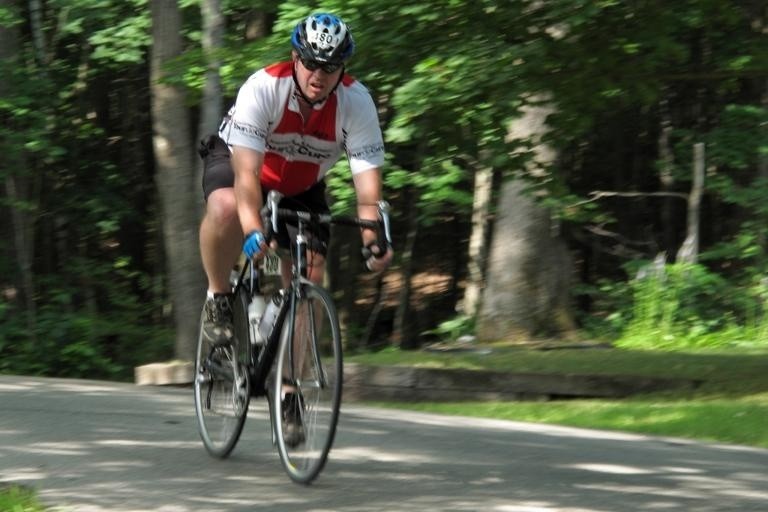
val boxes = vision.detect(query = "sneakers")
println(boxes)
[203,294,234,343]
[281,393,304,447]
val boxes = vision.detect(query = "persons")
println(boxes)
[195,15,395,445]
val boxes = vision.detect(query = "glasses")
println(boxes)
[298,56,343,73]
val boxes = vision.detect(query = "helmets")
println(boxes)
[291,13,353,61]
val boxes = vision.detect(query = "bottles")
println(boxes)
[247,288,287,344]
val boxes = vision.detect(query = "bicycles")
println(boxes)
[191,191,392,484]
